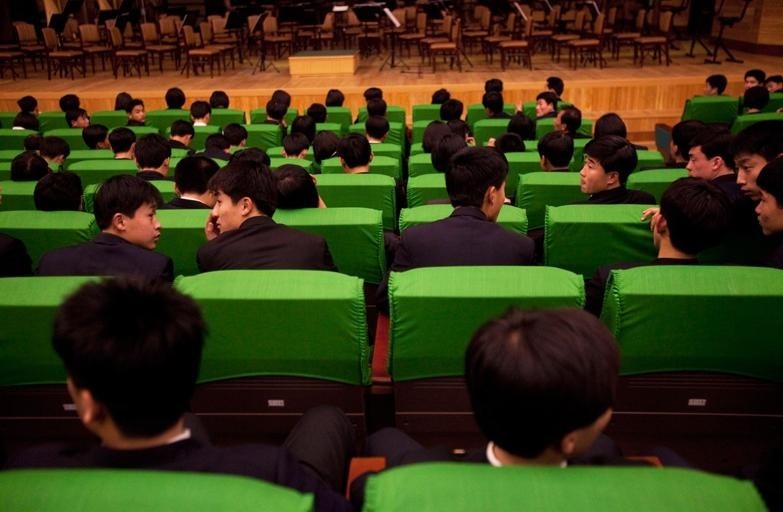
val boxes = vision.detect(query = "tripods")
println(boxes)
[226,33,253,67]
[252,40,280,76]
[354,21,412,71]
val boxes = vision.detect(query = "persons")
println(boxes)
[351,307,653,505]
[7,275,351,512]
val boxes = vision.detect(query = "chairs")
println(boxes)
[0,92,783,511]
[0,0,750,82]
